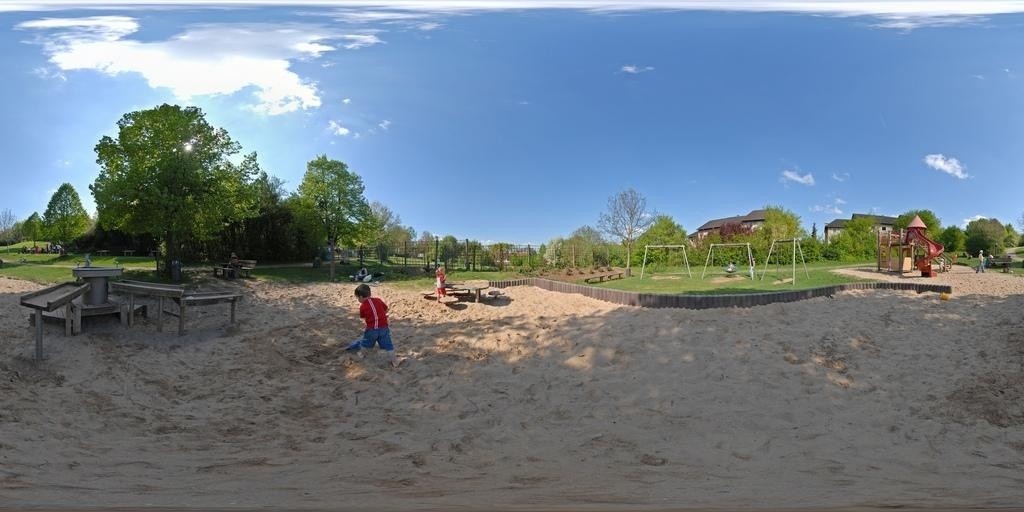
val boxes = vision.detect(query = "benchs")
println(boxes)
[607,269,624,281]
[96,250,110,256]
[584,272,605,284]
[121,247,136,256]
[150,248,160,257]
[238,260,257,278]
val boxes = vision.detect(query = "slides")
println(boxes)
[910,228,944,277]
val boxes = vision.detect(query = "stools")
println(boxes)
[420,291,434,298]
[440,297,459,306]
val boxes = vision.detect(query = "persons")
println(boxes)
[939,259,945,272]
[435,266,448,303]
[748,254,756,277]
[988,255,995,268]
[725,260,736,272]
[354,267,368,280]
[976,250,985,273]
[354,284,394,350]
[230,257,241,279]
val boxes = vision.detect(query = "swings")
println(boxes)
[718,242,739,273]
